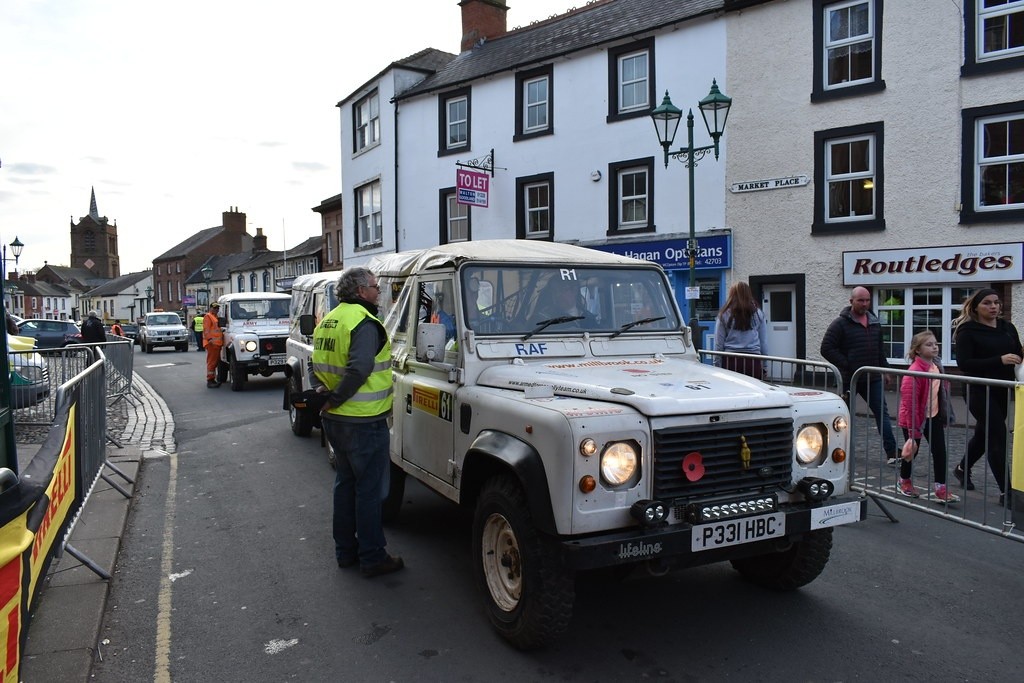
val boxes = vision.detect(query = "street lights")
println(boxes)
[0,236,24,328]
[9,282,19,315]
[145,286,155,312]
[649,77,733,363]
[201,261,214,313]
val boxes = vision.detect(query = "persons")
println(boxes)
[81,311,106,368]
[898,331,961,505]
[712,281,767,381]
[531,273,601,330]
[821,286,903,465]
[112,320,125,337]
[465,280,506,335]
[306,268,404,579]
[190,312,205,351]
[202,302,224,389]
[950,289,1024,509]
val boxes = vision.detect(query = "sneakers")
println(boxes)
[934,483,960,503]
[999,492,1012,510]
[953,464,974,490]
[897,476,919,497]
[886,448,902,464]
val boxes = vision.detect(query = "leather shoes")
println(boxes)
[360,556,404,578]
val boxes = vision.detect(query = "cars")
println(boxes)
[103,325,114,334]
[120,323,140,344]
[7,333,51,410]
[11,314,36,330]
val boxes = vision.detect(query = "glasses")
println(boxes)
[359,284,380,291]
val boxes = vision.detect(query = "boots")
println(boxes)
[211,379,221,386]
[207,379,219,387]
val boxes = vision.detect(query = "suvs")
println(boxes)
[365,239,869,653]
[15,319,85,357]
[282,271,344,469]
[215,291,292,391]
[139,312,189,353]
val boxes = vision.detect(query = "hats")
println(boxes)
[210,302,220,307]
